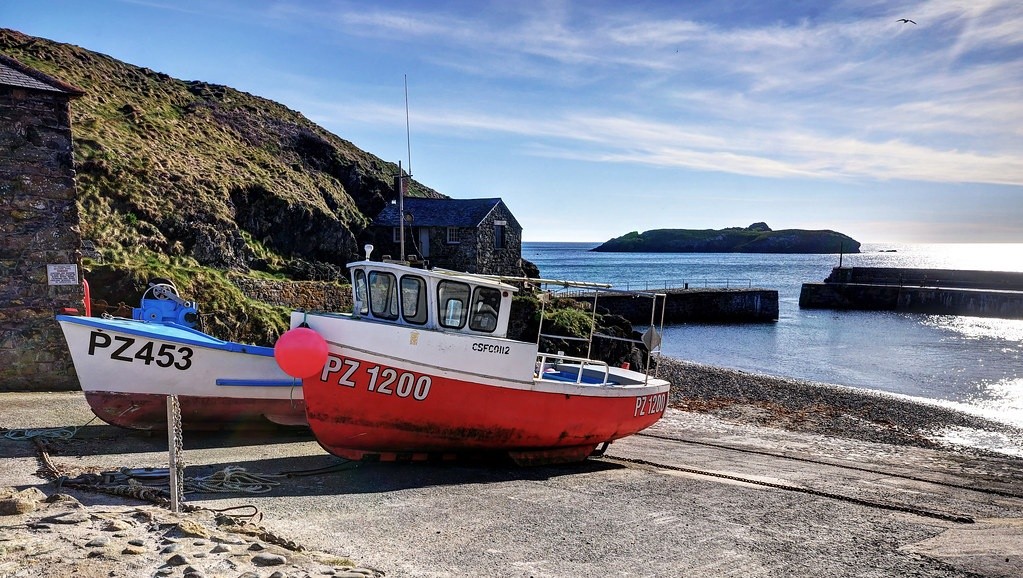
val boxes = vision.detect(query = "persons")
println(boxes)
[472,297,497,331]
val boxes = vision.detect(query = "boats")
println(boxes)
[56,277,312,434]
[273,73,671,465]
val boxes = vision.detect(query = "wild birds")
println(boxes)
[895,18,918,26]
